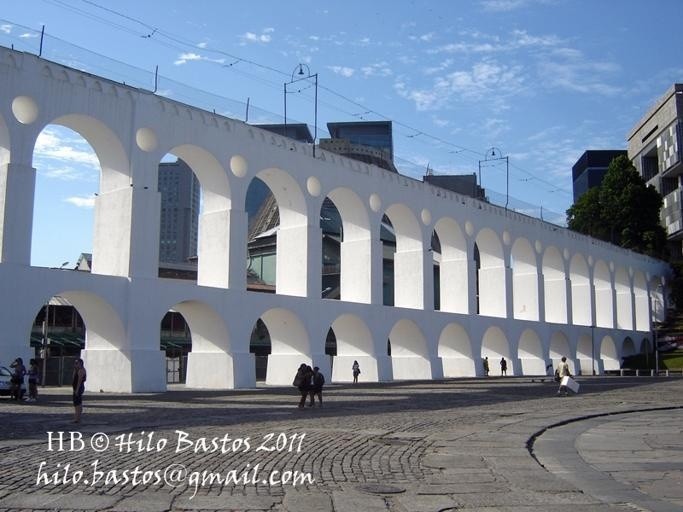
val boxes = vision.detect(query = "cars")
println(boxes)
[0,367,26,400]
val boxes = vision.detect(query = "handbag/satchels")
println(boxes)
[357,369,360,373]
[554,370,559,381]
[293,376,305,386]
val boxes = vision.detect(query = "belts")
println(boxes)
[561,376,579,393]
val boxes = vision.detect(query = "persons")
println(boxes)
[499,356,507,376]
[351,359,361,383]
[484,356,489,376]
[292,362,313,409]
[8,357,27,403]
[23,358,38,402]
[68,358,85,423]
[309,366,324,407]
[554,356,573,397]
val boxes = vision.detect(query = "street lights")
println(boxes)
[655,283,662,376]
[322,287,332,293]
[41,262,69,387]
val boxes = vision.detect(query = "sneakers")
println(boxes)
[558,390,562,394]
[24,398,37,402]
[565,392,569,395]
